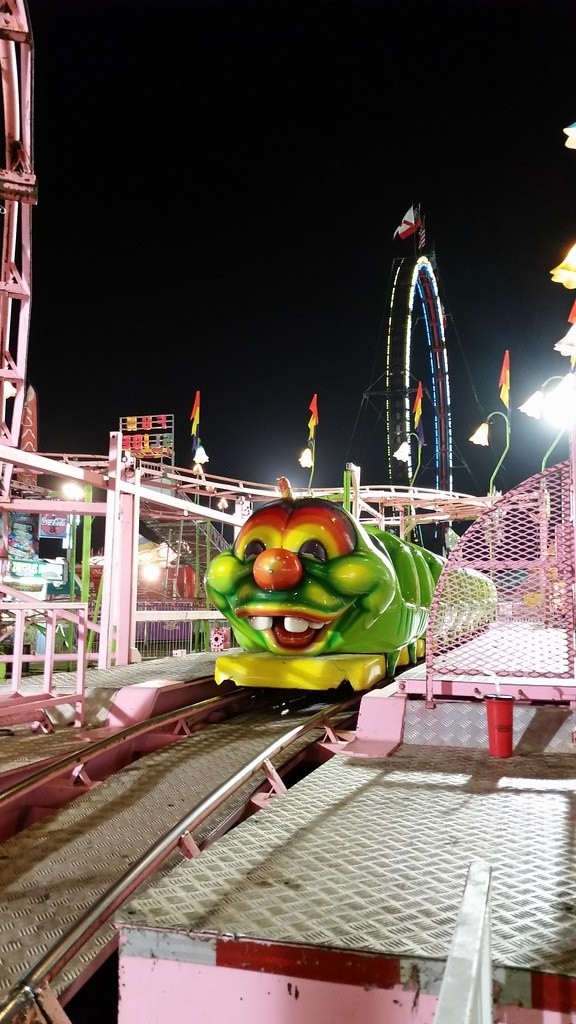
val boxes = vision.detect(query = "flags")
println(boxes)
[418,215,425,251]
[392,203,422,241]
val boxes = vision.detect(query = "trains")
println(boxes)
[204,466,497,695]
